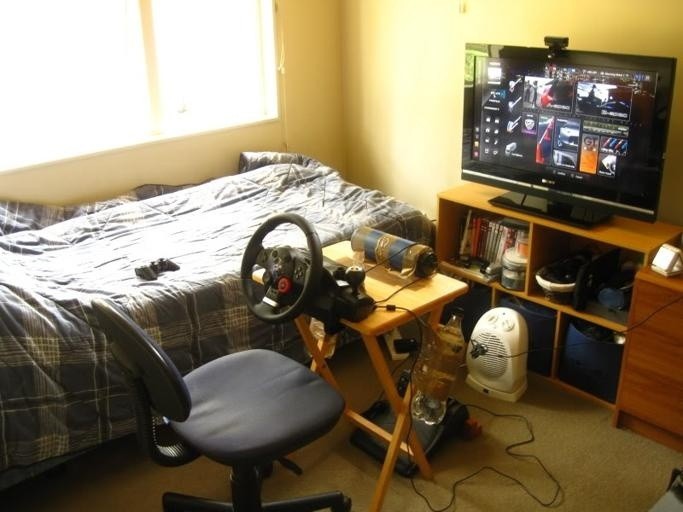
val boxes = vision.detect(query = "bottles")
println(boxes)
[409,308,465,429]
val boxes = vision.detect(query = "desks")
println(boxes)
[250,240,469,511]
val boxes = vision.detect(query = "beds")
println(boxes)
[2,164,432,489]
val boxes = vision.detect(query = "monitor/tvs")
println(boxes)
[461,44,677,229]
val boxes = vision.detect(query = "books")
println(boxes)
[455,209,527,269]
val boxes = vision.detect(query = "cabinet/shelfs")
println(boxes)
[612,266,683,450]
[436,180,683,413]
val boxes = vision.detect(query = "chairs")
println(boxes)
[90,298,354,511]
